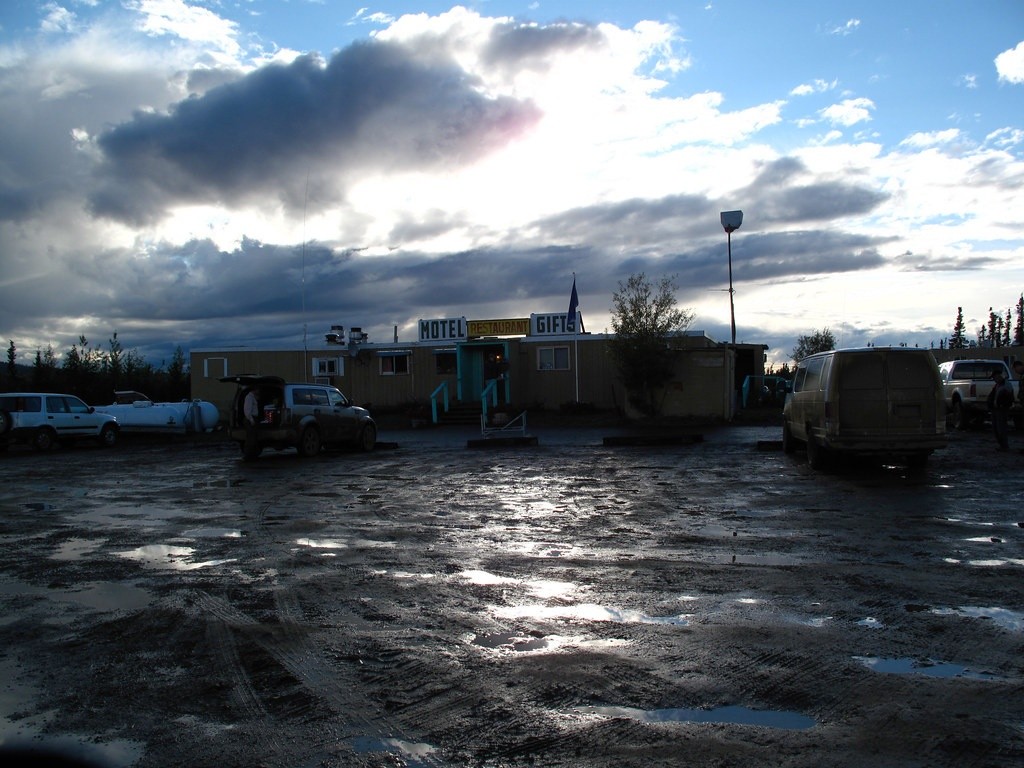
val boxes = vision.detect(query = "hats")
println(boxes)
[989,368,1002,379]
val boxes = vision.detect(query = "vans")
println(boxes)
[780,347,951,470]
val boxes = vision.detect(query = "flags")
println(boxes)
[567,279,579,332]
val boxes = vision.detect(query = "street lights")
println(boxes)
[721,210,745,345]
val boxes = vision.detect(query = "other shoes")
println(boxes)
[995,446,1009,452]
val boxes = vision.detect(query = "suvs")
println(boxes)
[743,376,789,404]
[0,391,121,451]
[214,374,378,458]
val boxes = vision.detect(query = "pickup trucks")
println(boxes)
[936,359,1020,430]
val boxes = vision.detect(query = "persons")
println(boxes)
[987,369,1014,451]
[446,360,456,373]
[243,384,263,462]
[127,397,134,402]
[437,363,443,373]
[115,395,120,402]
[484,353,497,379]
[1013,361,1024,452]
[16,397,26,410]
